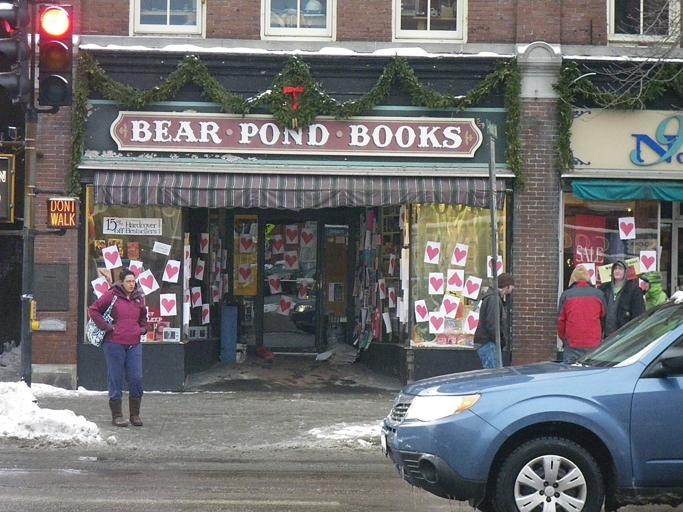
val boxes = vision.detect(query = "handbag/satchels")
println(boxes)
[86,313,114,347]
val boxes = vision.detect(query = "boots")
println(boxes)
[109,400,128,426]
[129,400,142,426]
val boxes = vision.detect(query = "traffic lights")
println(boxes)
[0,8,30,103]
[39,4,72,106]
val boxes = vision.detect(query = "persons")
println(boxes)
[640,271,669,311]
[88,268,148,426]
[556,265,606,365]
[598,260,646,339]
[473,272,514,368]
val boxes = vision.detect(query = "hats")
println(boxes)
[498,273,514,288]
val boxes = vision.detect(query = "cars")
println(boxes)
[381,297,683,512]
